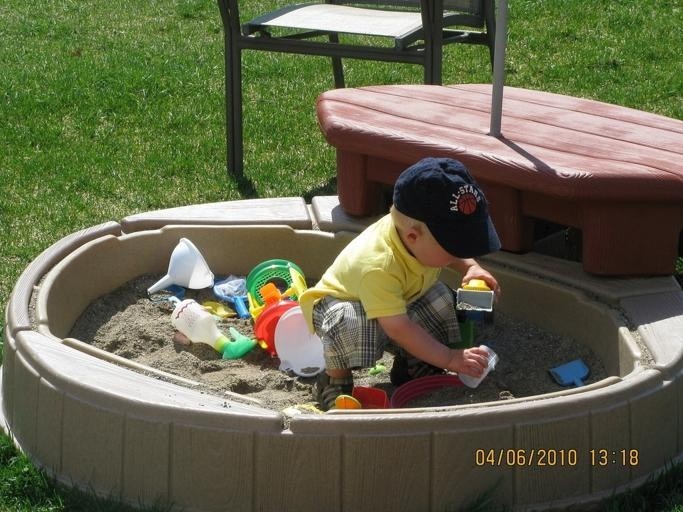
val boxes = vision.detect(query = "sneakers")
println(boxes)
[311,369,353,411]
[389,354,438,385]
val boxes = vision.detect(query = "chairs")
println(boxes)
[218,0,497,178]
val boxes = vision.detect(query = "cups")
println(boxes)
[457,343,500,389]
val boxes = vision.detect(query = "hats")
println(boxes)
[392,156,502,259]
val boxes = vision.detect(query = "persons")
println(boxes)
[295,156,500,408]
